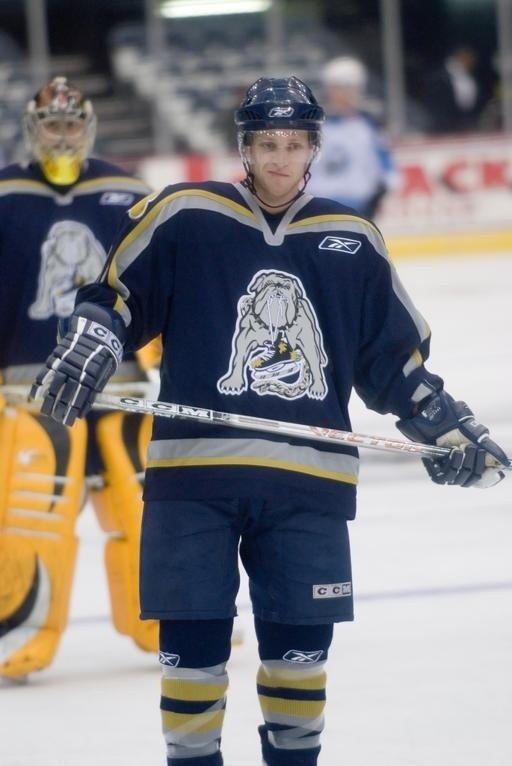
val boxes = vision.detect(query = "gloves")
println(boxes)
[27,301,126,427]
[395,390,510,490]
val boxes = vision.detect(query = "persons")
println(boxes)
[298,54,403,220]
[27,76,509,765]
[0,76,160,686]
[421,40,492,131]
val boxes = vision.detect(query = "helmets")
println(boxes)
[233,76,325,148]
[23,77,97,127]
